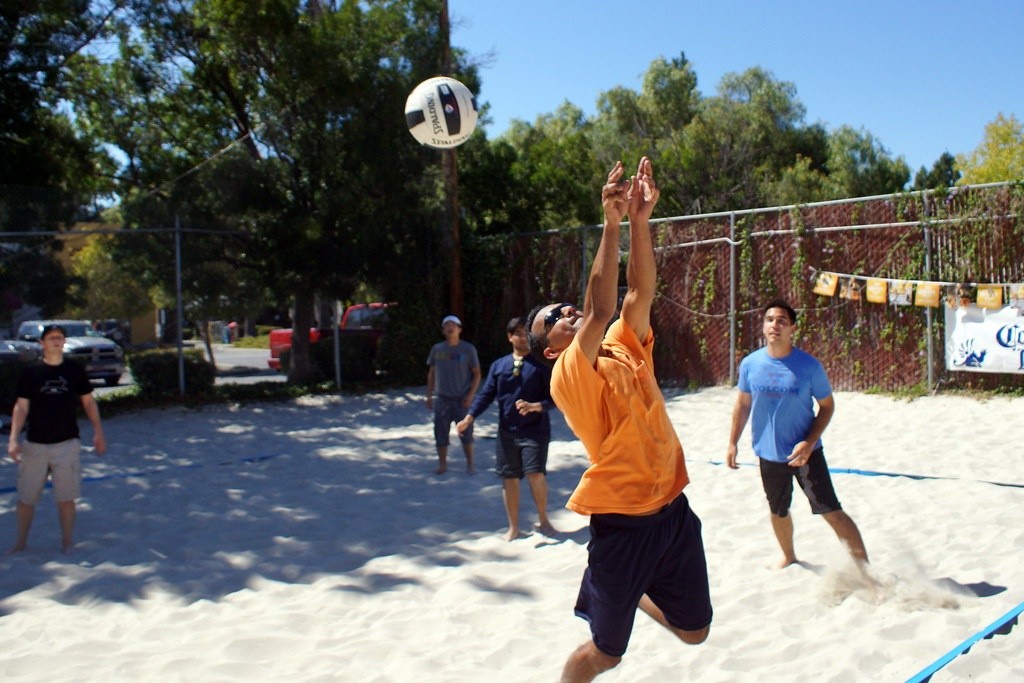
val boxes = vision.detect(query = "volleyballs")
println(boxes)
[405,75,479,149]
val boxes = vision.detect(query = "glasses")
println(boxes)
[544,301,578,349]
[512,360,523,379]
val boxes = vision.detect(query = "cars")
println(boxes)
[0,340,40,371]
[93,318,124,344]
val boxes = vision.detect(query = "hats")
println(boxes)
[41,325,66,340]
[441,316,462,328]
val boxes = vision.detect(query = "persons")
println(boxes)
[726,299,869,567]
[456,316,556,541]
[424,315,481,474]
[8,323,107,553]
[525,157,714,683]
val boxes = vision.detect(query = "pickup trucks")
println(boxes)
[269,302,397,372]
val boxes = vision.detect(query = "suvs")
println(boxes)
[17,320,125,386]
